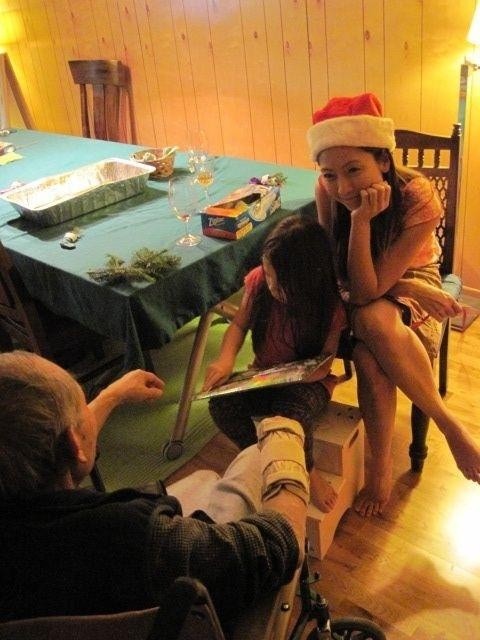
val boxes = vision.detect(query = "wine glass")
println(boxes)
[167,176,202,248]
[192,157,216,215]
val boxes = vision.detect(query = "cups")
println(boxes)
[184,145,208,173]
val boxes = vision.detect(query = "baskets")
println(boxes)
[132,148,176,179]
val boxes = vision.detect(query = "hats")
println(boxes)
[308,93,397,163]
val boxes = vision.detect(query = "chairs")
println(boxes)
[1,244,127,492]
[69,60,126,141]
[1,535,392,640]
[394,123,461,401]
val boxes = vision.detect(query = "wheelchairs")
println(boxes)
[0,445,386,640]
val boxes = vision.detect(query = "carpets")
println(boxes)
[80,306,255,494]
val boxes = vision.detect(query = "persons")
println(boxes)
[309,95,480,519]
[203,214,346,514]
[0,350,311,638]
